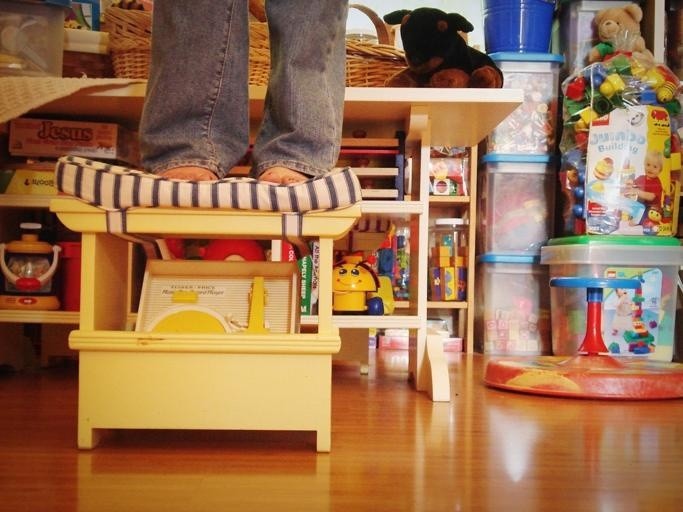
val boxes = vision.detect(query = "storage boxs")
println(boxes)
[540,236,683,363]
[1,1,72,77]
[559,1,630,78]
[474,51,565,360]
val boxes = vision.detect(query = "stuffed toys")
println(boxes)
[383,8,503,88]
[588,4,656,64]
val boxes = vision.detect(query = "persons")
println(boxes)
[613,288,632,335]
[625,154,662,228]
[139,0,348,184]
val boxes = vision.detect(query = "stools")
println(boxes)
[49,193,362,454]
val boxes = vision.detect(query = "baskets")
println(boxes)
[104,0,408,88]
[104,1,408,88]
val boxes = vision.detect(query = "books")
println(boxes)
[378,319,463,353]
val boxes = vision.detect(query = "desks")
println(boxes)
[0,76,525,402]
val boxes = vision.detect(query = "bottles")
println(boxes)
[393,217,470,301]
[4,234,61,294]
